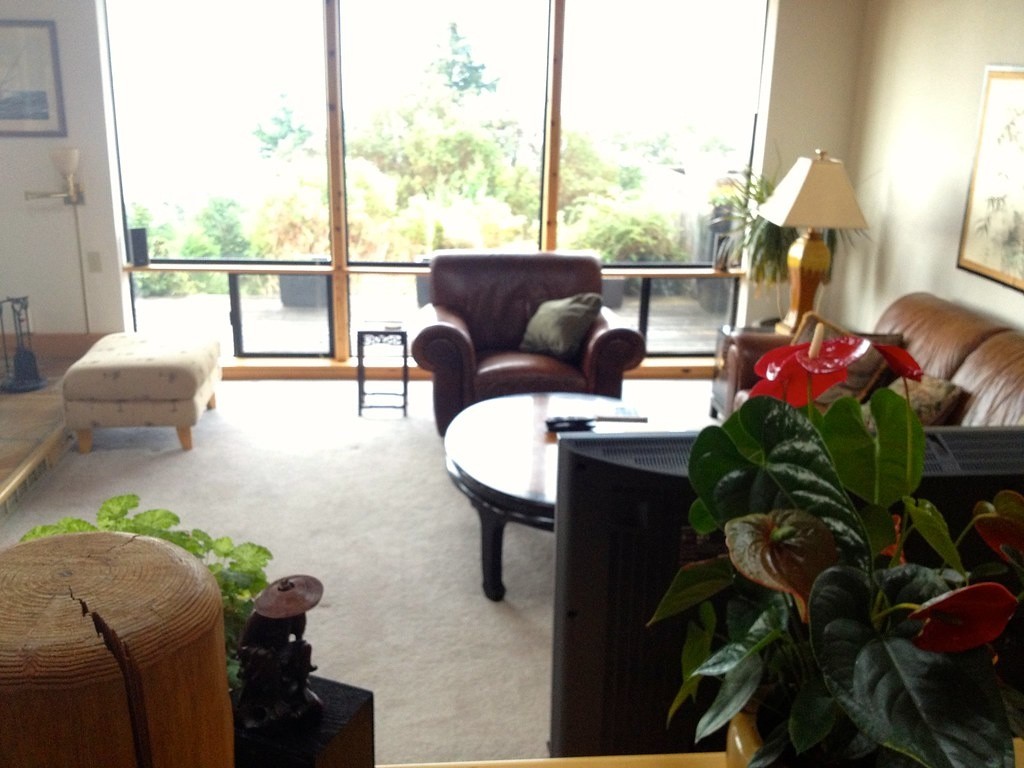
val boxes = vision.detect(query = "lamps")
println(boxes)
[763,149,867,335]
[50,146,79,201]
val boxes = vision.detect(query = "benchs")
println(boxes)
[64,331,222,451]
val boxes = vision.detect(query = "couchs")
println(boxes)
[731,292,1024,428]
[416,254,645,437]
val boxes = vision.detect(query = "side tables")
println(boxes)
[356,321,408,413]
[709,326,775,417]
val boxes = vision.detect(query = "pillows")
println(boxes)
[859,373,971,429]
[521,293,600,358]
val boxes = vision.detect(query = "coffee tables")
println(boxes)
[445,392,652,603]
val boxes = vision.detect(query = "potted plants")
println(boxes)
[719,167,853,327]
[265,196,373,306]
[647,386,1024,767]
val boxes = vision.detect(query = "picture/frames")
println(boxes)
[954,64,1024,292]
[0,19,66,138]
